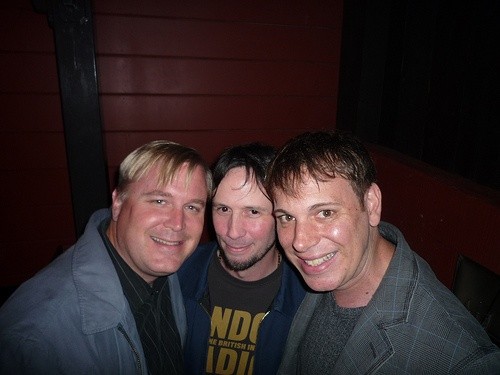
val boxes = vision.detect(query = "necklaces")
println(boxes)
[218,246,281,267]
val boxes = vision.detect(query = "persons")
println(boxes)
[178,142,309,375]
[0,139,217,375]
[267,132,500,375]
